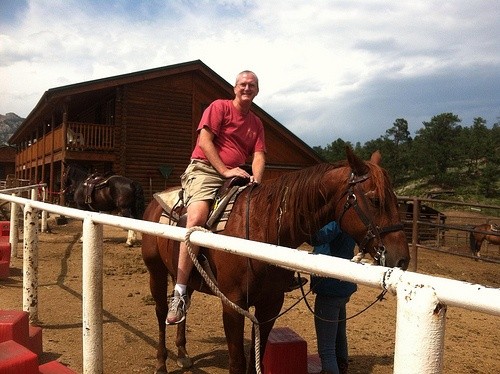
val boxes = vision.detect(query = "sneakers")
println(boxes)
[165,290,191,325]
[285,277,308,292]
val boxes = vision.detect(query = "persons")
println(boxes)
[305,219,357,374]
[165,71,267,324]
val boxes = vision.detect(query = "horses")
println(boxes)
[141,145,411,374]
[60,160,145,247]
[469,224,500,262]
